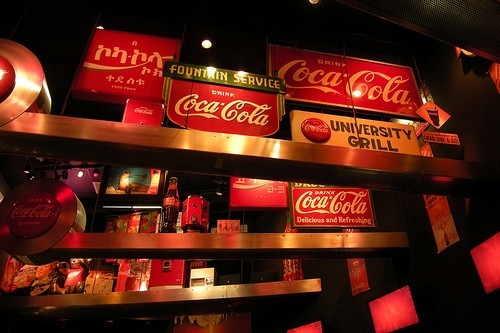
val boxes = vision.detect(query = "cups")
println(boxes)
[427,109,440,127]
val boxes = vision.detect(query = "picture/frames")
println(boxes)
[267,44,424,122]
[96,164,165,206]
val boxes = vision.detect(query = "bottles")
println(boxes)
[161,177,181,233]
[114,259,130,292]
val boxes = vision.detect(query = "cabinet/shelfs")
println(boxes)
[0,38,482,323]
